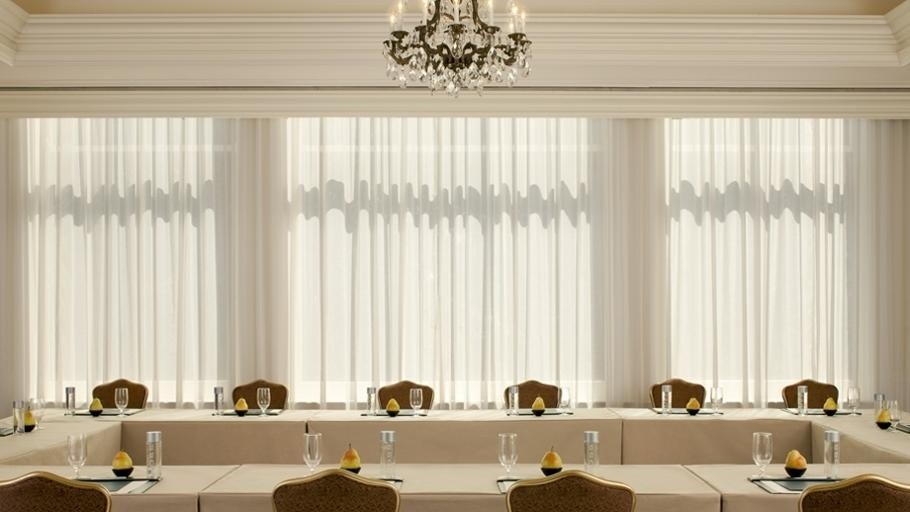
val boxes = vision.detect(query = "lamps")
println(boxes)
[382,0,533,97]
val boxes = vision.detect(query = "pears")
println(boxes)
[90,397,103,410]
[532,396,544,410]
[824,396,837,410]
[340,442,361,469]
[541,446,562,469]
[23,406,35,425]
[687,396,700,410]
[785,449,808,469]
[113,449,133,469]
[878,409,890,422]
[386,397,400,411]
[234,396,248,410]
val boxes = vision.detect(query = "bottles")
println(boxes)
[145,431,162,481]
[661,385,672,413]
[367,386,378,417]
[65,387,75,416]
[380,431,396,480]
[822,430,840,478]
[508,386,520,417]
[214,387,223,416]
[798,386,808,416]
[12,401,24,435]
[584,431,599,476]
[874,392,885,419]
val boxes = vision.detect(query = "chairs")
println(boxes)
[231,380,289,408]
[782,380,839,410]
[650,378,706,409]
[92,380,148,409]
[505,380,562,408]
[379,381,434,409]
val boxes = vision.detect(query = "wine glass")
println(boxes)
[709,387,724,416]
[302,433,323,476]
[751,431,773,479]
[65,432,87,479]
[256,388,271,417]
[114,388,129,418]
[409,388,423,418]
[497,432,520,484]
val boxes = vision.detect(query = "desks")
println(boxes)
[0,410,908,511]
